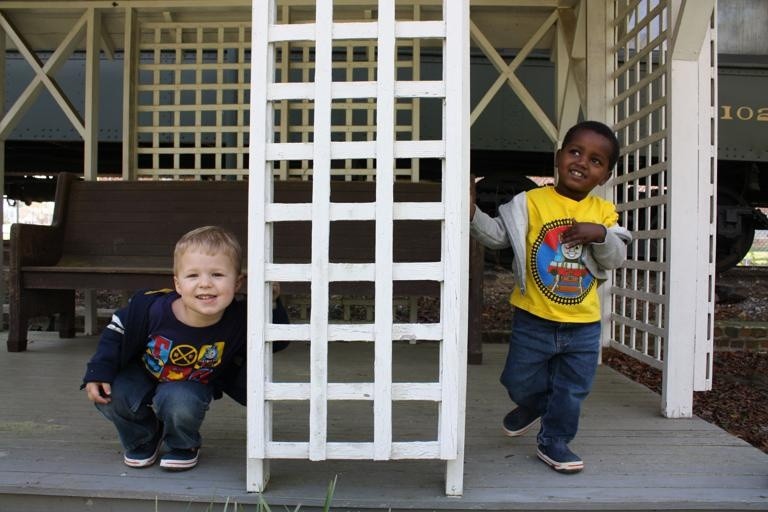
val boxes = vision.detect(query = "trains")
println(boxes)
[0,0,768,272]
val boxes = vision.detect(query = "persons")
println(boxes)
[79,225,290,469]
[470,121,633,472]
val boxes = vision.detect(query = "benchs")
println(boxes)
[8,173,483,364]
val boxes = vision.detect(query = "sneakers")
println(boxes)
[160,447,200,470]
[502,408,539,436]
[124,420,164,467]
[537,444,584,471]
